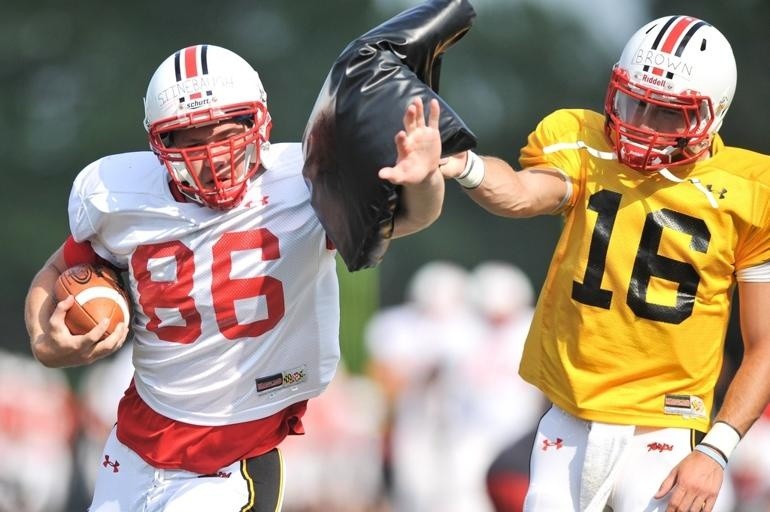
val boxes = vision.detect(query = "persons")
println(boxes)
[438,14,770,511]
[697,392,769,511]
[0,337,134,512]
[28,43,444,512]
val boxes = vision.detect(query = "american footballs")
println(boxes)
[52,262,132,348]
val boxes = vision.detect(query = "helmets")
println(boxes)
[143,44,272,165]
[604,14,738,145]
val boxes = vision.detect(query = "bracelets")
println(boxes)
[455,147,487,191]
